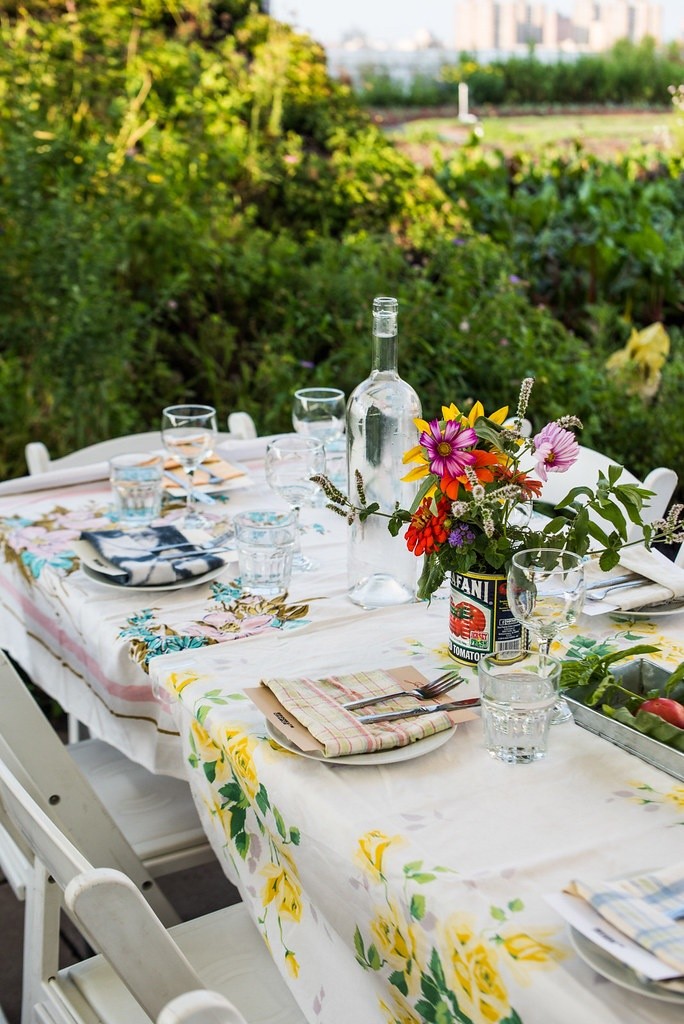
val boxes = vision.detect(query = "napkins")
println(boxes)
[562,862,684,997]
[144,441,245,490]
[259,668,453,758]
[80,523,225,586]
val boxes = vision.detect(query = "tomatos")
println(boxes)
[635,697,684,729]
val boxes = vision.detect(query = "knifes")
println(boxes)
[165,469,216,505]
[157,544,237,562]
[357,697,481,725]
[537,572,646,596]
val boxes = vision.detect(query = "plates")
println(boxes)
[264,714,457,765]
[559,657,684,783]
[563,923,684,1004]
[79,556,231,593]
[562,556,684,619]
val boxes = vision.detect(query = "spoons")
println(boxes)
[198,462,224,484]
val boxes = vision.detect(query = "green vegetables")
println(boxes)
[548,643,684,754]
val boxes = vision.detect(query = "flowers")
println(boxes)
[308,376,683,617]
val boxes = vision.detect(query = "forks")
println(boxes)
[341,670,465,710]
[150,528,234,553]
[587,578,655,601]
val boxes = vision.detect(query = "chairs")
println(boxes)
[0,740,310,1023]
[502,416,678,546]
[1,652,217,932]
[24,411,257,476]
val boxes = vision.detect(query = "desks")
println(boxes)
[1,432,684,1024]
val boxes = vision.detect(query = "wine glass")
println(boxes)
[263,436,327,574]
[292,386,346,509]
[506,547,586,726]
[160,404,218,531]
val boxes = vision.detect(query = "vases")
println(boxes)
[448,568,529,664]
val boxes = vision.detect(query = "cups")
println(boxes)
[109,451,164,528]
[477,649,562,765]
[232,507,297,602]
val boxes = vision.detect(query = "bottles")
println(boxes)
[345,296,425,610]
